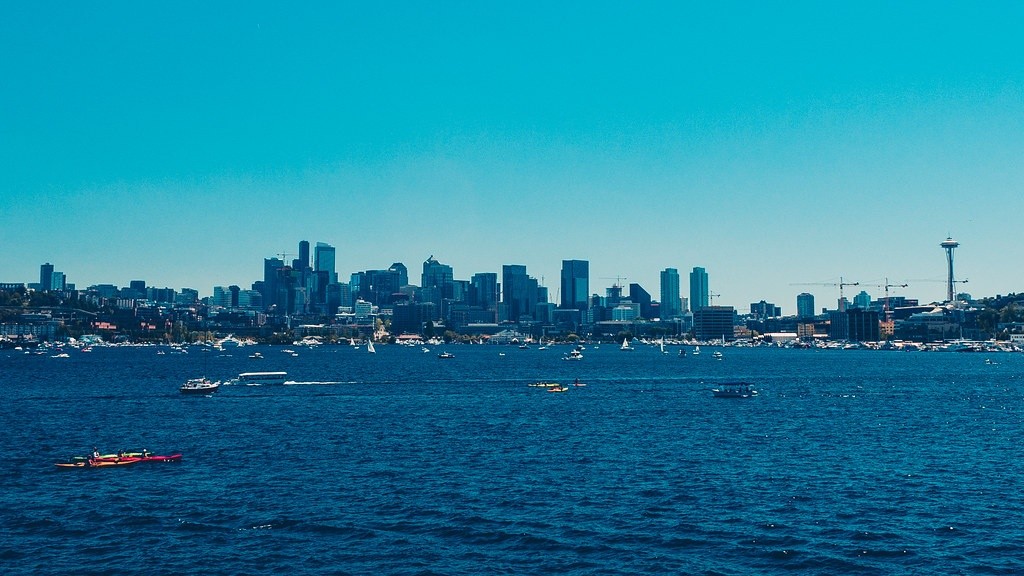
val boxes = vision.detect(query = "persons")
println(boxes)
[141,449,146,457]
[117,449,123,457]
[87,455,93,464]
[93,446,99,457]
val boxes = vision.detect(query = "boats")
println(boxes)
[547,387,568,392]
[678,346,723,358]
[712,382,758,398]
[54,451,183,467]
[528,384,560,387]
[568,383,586,387]
[231,372,288,385]
[15,346,93,358]
[157,334,324,358]
[437,354,455,358]
[519,344,585,360]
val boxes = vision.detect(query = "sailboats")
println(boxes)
[179,322,221,393]
[367,339,376,353]
[621,338,629,349]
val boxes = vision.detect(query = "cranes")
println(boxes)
[823,277,908,322]
[708,290,720,306]
[905,273,968,300]
[277,251,299,263]
[789,277,860,312]
[596,275,627,298]
